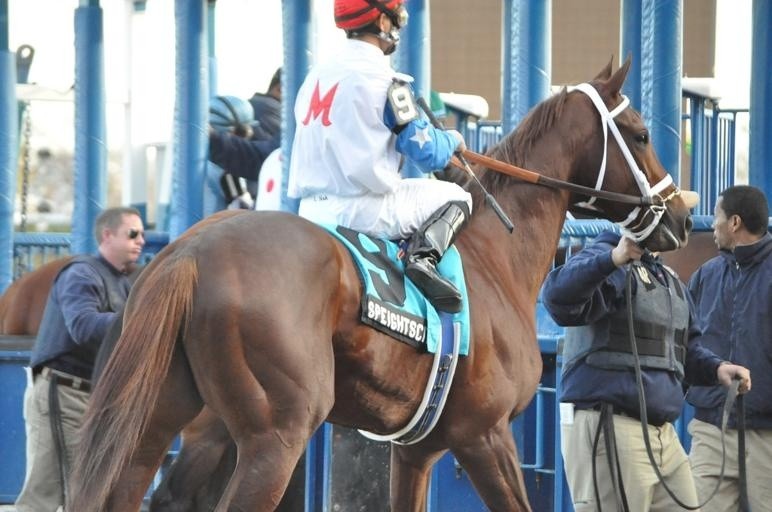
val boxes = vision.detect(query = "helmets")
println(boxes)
[334,0,404,30]
[210,93,260,130]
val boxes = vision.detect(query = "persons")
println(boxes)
[249,65,282,198]
[286,0,473,315]
[540,221,752,512]
[12,207,146,512]
[203,95,260,215]
[685,185,772,512]
[209,126,282,181]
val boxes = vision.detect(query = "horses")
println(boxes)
[1,253,141,338]
[65,51,696,511]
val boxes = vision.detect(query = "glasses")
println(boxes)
[126,228,144,239]
[390,6,409,27]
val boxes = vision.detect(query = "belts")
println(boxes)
[46,372,91,392]
[592,404,655,425]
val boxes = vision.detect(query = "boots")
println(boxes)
[403,202,466,314]
